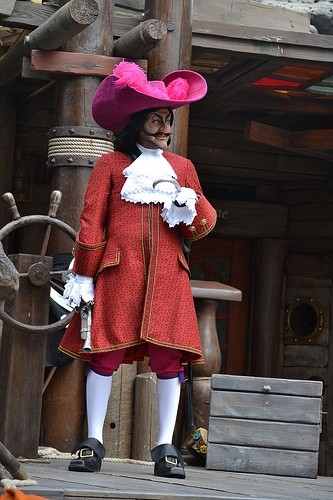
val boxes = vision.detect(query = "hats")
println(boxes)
[91,63,208,130]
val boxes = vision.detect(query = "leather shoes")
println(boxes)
[151,444,186,479]
[68,438,106,472]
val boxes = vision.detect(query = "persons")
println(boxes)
[59,61,218,480]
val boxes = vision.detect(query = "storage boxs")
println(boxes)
[205,373,322,479]
